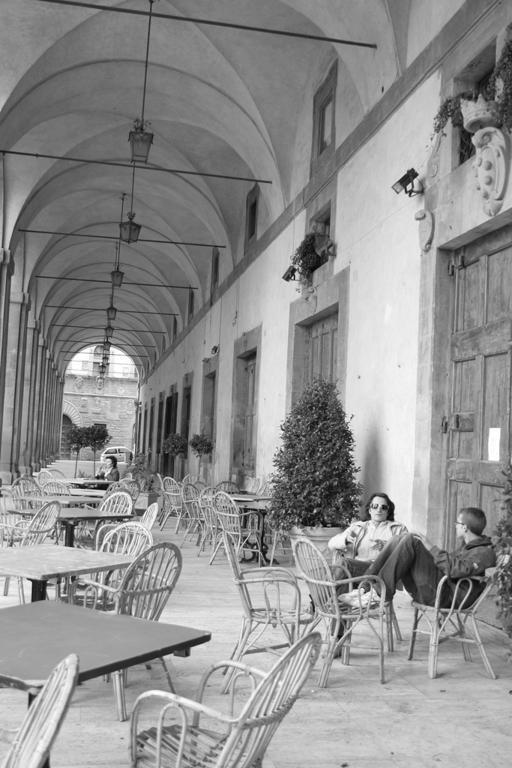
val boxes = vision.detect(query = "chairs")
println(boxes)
[1,445,281,565]
[107,541,186,725]
[332,550,402,652]
[221,531,316,695]
[408,556,503,680]
[291,538,388,687]
[1,650,82,767]
[125,630,326,768]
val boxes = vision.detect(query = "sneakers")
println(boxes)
[336,587,365,603]
[349,587,390,607]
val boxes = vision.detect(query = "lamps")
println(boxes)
[126,0,155,165]
[118,161,144,244]
[390,167,425,199]
[281,264,300,283]
[210,345,220,354]
[92,191,129,389]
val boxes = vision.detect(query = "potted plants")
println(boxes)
[263,377,369,582]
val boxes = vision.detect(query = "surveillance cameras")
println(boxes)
[392,168,419,195]
[283,266,297,282]
[211,346,217,354]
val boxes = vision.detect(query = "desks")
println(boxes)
[1,598,213,767]
[2,536,138,606]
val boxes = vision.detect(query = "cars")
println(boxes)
[99,445,133,461]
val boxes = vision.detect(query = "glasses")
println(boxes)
[369,502,389,509]
[455,520,464,526]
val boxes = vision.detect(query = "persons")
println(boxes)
[96,456,119,490]
[306,492,408,658]
[337,508,497,609]
